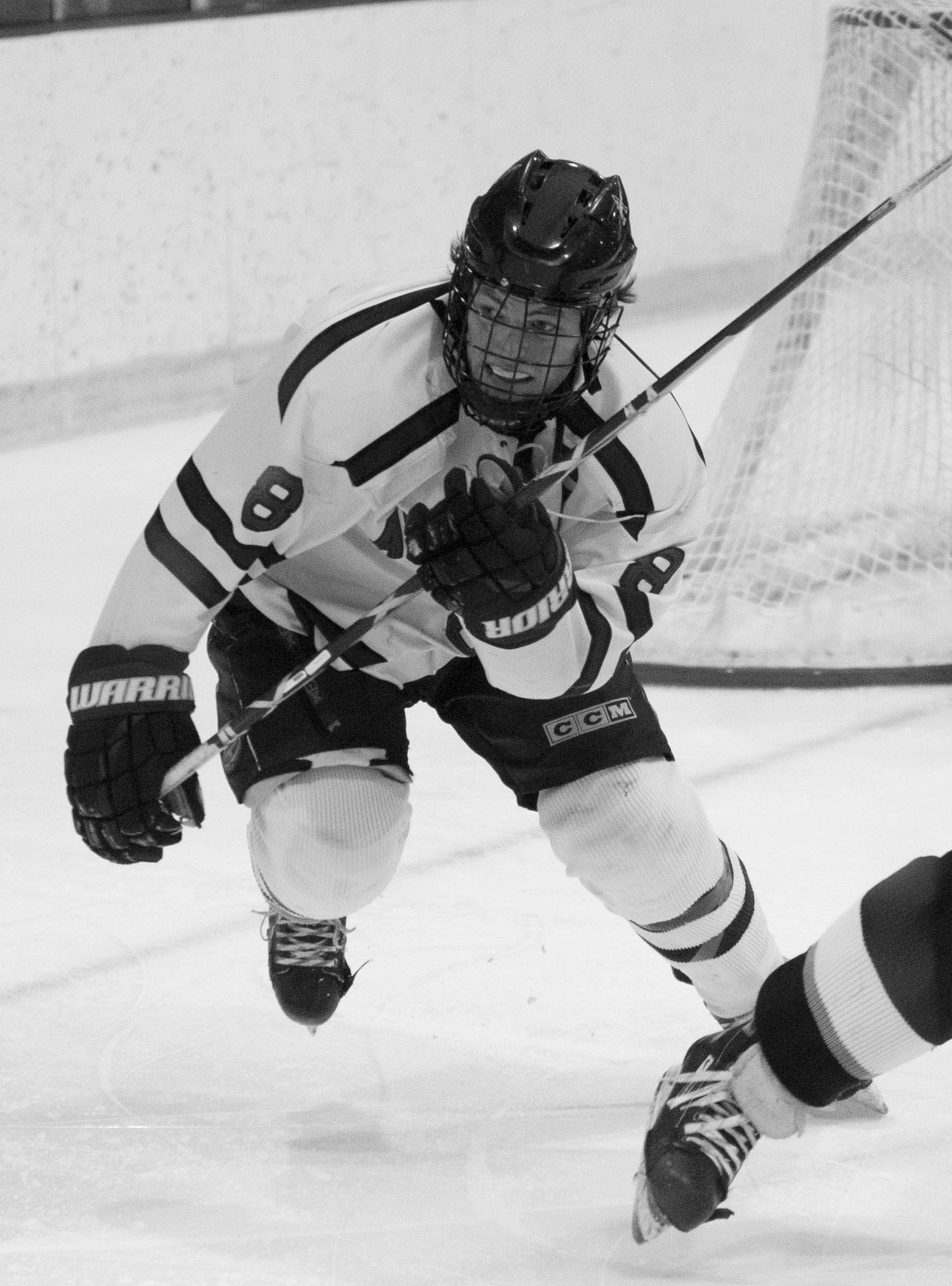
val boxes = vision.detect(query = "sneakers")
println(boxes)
[631,1019,807,1245]
[252,905,371,1039]
[670,964,890,1127]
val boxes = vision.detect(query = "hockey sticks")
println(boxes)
[156,154,952,799]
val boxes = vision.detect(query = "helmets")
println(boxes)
[440,149,643,433]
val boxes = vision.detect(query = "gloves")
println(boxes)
[64,643,205,865]
[405,453,579,649]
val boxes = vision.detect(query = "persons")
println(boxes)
[628,850,952,1244]
[63,149,886,1120]
